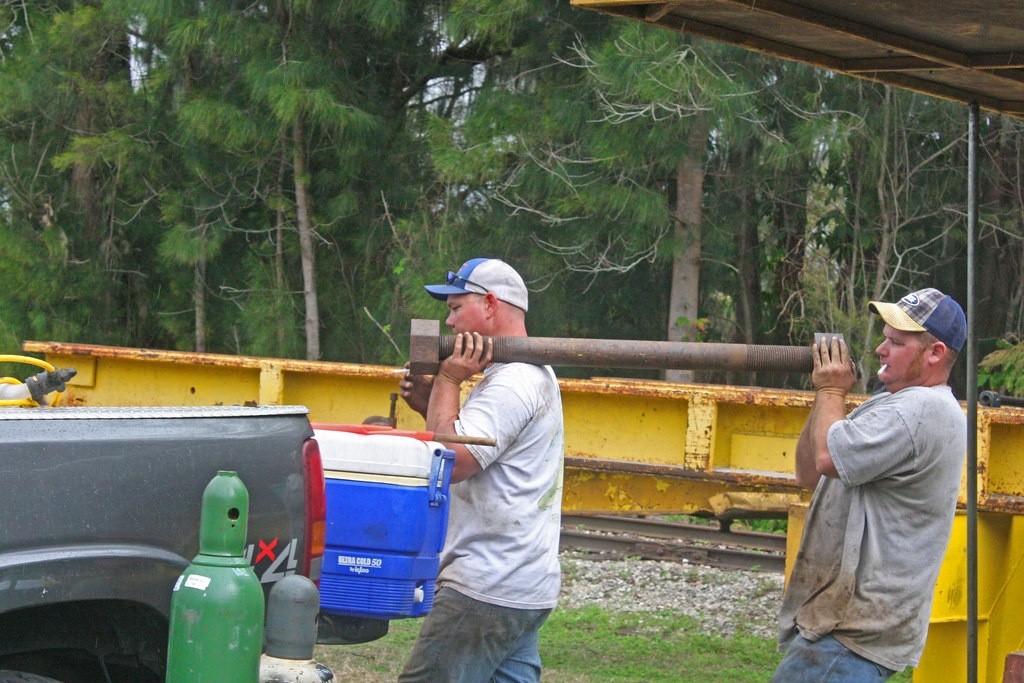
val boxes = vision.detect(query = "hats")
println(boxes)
[424,257,528,312]
[867,288,967,353]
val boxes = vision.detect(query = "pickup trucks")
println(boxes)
[0,400,389,682]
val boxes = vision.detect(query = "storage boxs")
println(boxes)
[313,428,455,617]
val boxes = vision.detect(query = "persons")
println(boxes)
[768,289,968,682]
[397,259,564,683]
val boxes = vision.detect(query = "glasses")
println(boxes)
[447,270,488,291]
